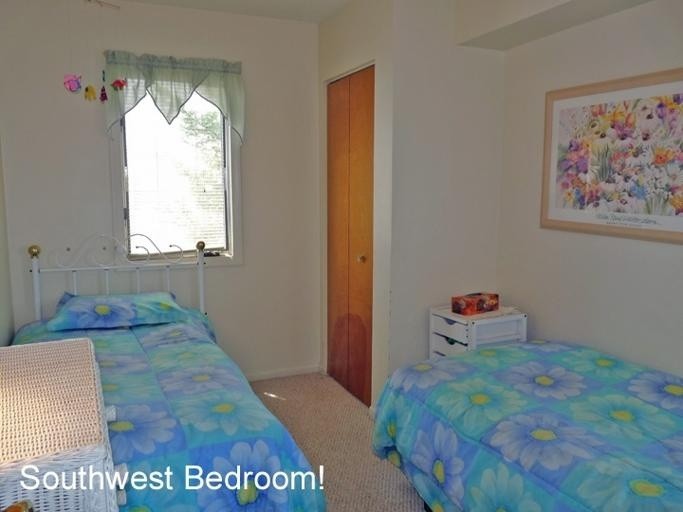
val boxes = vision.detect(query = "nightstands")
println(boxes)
[428,303,529,364]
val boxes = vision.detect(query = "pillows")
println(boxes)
[45,290,187,332]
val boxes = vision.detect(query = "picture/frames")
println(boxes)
[540,68,683,246]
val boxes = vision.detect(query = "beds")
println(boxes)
[367,338,683,511]
[10,233,329,512]
